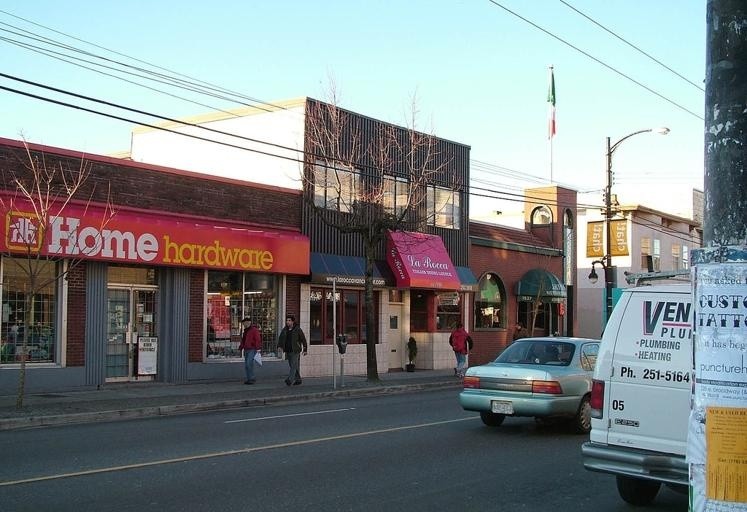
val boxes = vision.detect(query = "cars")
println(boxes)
[459,335,600,433]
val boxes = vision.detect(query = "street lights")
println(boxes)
[588,126,671,316]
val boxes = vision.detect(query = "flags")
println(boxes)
[547,67,556,137]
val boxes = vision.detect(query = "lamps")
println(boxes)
[588,260,605,284]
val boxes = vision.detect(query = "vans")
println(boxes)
[579,280,694,507]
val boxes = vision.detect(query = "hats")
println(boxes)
[242,317,250,322]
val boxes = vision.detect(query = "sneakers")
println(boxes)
[454,367,459,374]
[293,382,302,385]
[459,373,464,377]
[244,380,256,384]
[285,378,291,385]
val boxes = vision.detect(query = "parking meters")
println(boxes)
[337,335,347,389]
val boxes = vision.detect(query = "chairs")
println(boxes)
[555,351,571,362]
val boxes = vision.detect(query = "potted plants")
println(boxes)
[404,337,417,372]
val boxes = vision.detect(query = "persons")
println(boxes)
[513,322,529,341]
[277,315,307,386]
[449,324,473,377]
[239,318,261,385]
